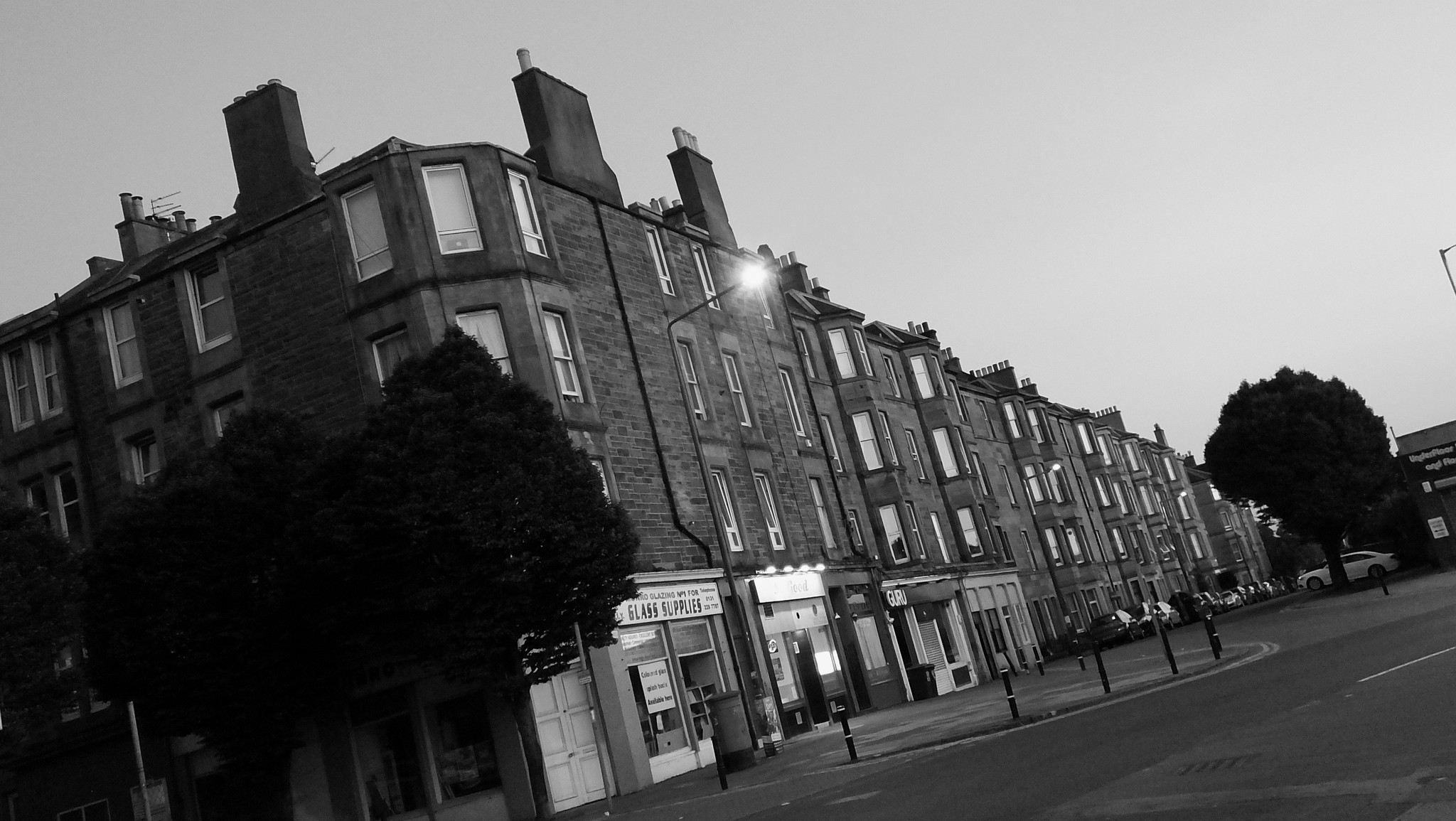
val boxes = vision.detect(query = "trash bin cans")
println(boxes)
[906,663,936,701]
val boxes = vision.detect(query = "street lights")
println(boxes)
[666,264,784,756]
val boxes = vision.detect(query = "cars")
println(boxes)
[1243,575,1298,603]
[1124,602,1157,637]
[1297,551,1400,591]
[1228,586,1255,605]
[1193,593,1211,620]
[1199,592,1219,615]
[1152,602,1183,633]
[1211,592,1226,614]
[1220,591,1244,611]
[1090,609,1144,652]
